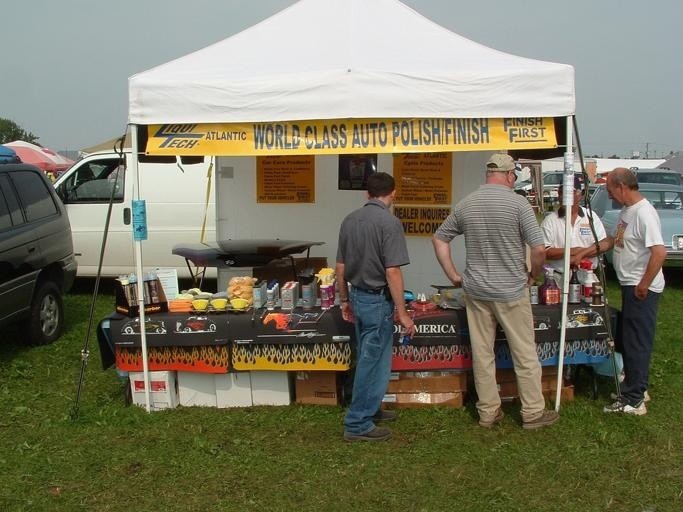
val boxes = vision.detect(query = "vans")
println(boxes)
[50,149,216,282]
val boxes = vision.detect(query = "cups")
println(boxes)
[210,299,226,309]
[230,299,248,309]
[432,294,440,305]
[191,299,209,310]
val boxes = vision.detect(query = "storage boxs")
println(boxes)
[495,368,574,404]
[177,371,216,408]
[251,370,291,407]
[216,371,253,409]
[381,370,467,409]
[128,370,178,409]
[294,369,342,407]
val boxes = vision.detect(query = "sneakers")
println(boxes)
[478,408,506,428]
[342,426,392,443]
[520,409,560,430]
[370,408,397,422]
[611,389,652,402]
[603,399,649,418]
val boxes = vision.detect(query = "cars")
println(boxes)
[584,167,682,274]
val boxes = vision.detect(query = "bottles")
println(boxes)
[539,267,560,305]
[530,282,539,305]
[578,260,602,305]
[568,268,580,304]
[252,279,335,310]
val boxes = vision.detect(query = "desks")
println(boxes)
[91,291,616,410]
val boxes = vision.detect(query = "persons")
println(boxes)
[570,167,667,415]
[430,153,560,429]
[336,173,416,441]
[538,177,613,290]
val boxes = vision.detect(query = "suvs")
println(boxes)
[0,163,78,344]
[515,168,588,196]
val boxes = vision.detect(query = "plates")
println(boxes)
[588,303,604,307]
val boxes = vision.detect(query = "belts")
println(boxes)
[350,284,386,295]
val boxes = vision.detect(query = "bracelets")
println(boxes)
[342,297,349,302]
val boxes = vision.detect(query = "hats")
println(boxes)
[486,152,521,174]
